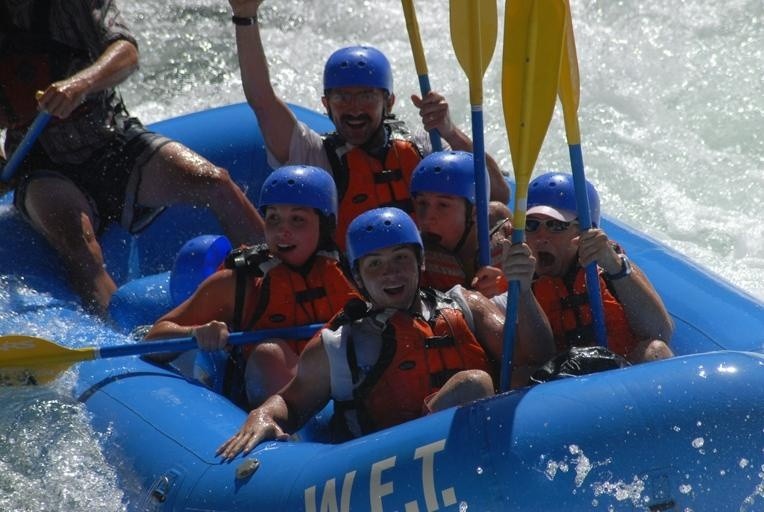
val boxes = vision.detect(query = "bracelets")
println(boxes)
[229,12,261,27]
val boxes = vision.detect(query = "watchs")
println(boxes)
[596,252,631,282]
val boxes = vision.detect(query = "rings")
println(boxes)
[428,113,439,122]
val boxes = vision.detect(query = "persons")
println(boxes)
[134,161,366,424]
[404,146,512,292]
[490,169,679,393]
[227,0,516,252]
[0,1,272,319]
[210,206,555,463]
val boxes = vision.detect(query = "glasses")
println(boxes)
[325,92,388,105]
[526,216,579,234]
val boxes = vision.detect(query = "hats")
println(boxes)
[526,206,578,223]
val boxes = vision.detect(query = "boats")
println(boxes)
[0,101,763,511]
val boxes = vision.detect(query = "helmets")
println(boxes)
[407,150,490,218]
[321,47,394,100]
[258,164,337,230]
[525,171,601,231]
[345,206,424,274]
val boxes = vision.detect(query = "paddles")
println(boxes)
[402,1,442,151]
[449,0,497,269]
[500,0,567,392]
[1,324,327,388]
[557,1,608,350]
